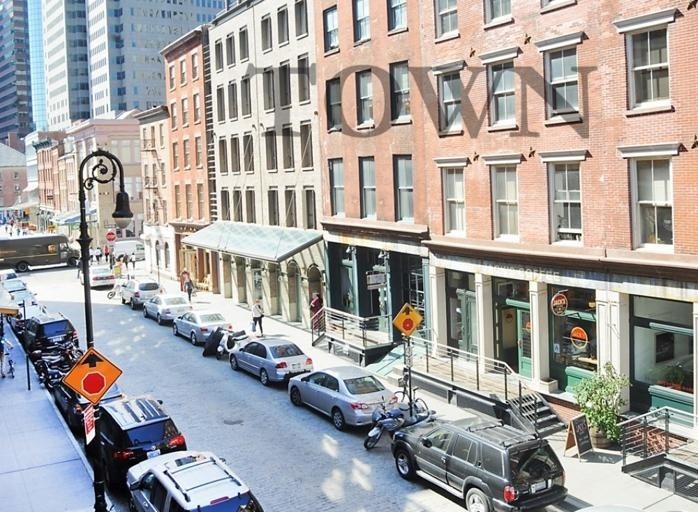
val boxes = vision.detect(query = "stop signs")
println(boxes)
[105,232,115,241]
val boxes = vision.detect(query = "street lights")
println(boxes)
[78,148,140,512]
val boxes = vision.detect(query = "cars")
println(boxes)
[285,365,397,432]
[661,355,697,394]
[80,266,319,391]
[0,268,80,360]
[52,369,128,432]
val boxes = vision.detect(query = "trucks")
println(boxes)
[0,234,80,274]
[113,239,145,263]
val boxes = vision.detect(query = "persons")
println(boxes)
[252,299,263,337]
[0,332,12,378]
[90,245,136,270]
[184,273,195,304]
[5,219,27,236]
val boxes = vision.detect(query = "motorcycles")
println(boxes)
[25,329,86,390]
[365,392,440,456]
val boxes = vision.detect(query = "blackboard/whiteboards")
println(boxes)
[571,415,593,457]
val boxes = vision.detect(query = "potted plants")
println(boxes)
[573,362,632,449]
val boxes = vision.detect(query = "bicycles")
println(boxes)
[394,374,430,420]
[1,346,14,379]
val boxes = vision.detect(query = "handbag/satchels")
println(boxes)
[250,322,256,331]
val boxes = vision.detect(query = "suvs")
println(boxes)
[72,394,190,493]
[121,450,269,512]
[386,412,568,512]
